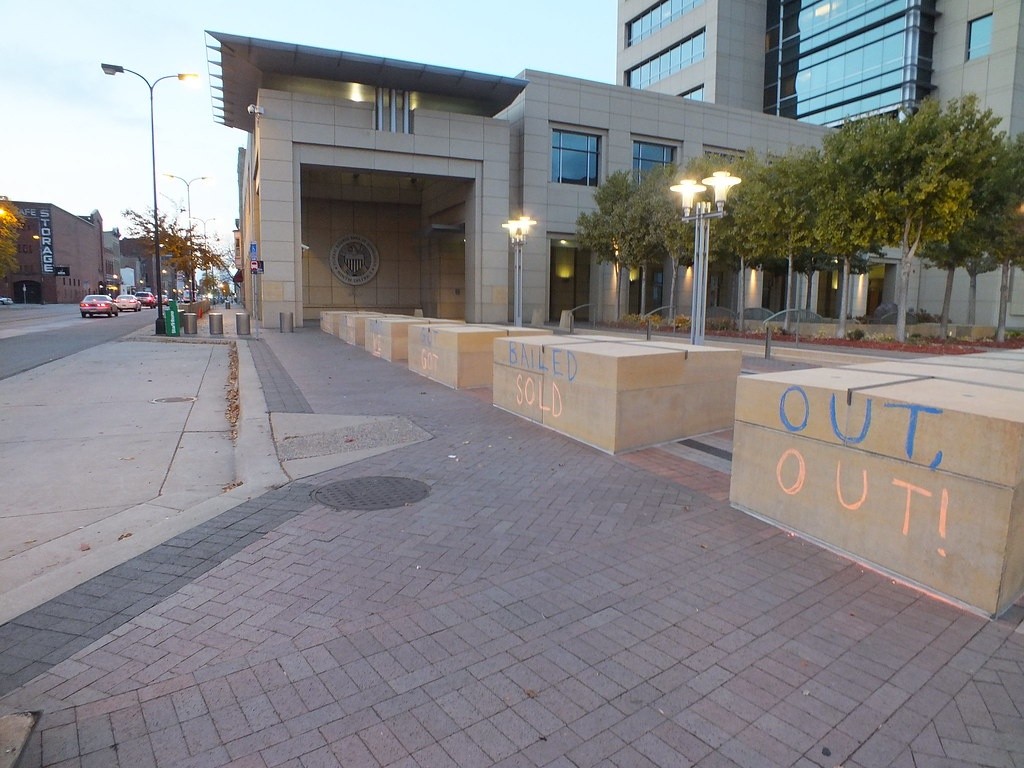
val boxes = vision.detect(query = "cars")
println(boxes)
[115,294,141,312]
[0,295,13,305]
[177,296,184,303]
[79,294,119,318]
[155,295,168,305]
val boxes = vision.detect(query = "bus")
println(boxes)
[183,290,197,303]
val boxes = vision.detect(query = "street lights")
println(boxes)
[669,170,742,346]
[168,173,205,306]
[193,216,216,248]
[500,216,538,328]
[101,62,198,335]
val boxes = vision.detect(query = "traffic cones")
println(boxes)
[198,307,203,319]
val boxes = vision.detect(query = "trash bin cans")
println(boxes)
[164,300,180,337]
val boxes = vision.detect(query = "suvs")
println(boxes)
[135,292,156,309]
